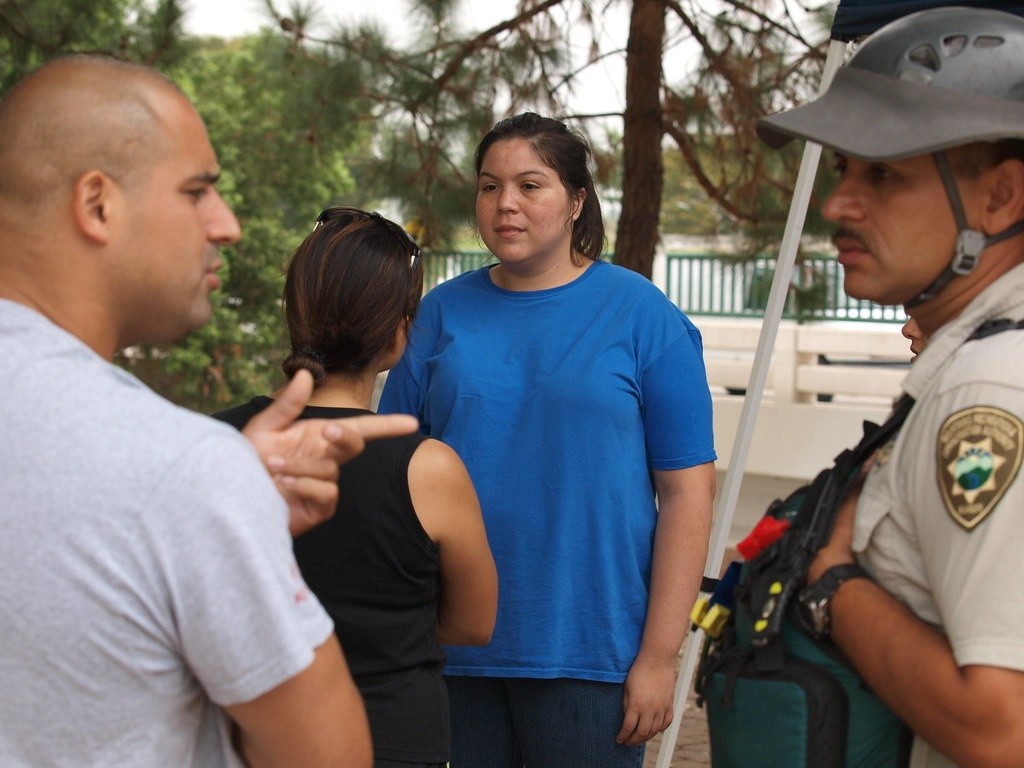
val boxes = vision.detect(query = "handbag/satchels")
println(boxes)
[693,464,913,767]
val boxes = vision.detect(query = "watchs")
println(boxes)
[796,561,876,642]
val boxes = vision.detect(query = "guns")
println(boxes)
[699,391,917,643]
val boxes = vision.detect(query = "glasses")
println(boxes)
[313,206,423,273]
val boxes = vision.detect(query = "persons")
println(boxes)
[374,106,719,768]
[1,45,418,768]
[186,196,500,768]
[900,301,930,366]
[781,1,1024,768]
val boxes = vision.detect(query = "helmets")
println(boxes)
[757,4,1023,164]
